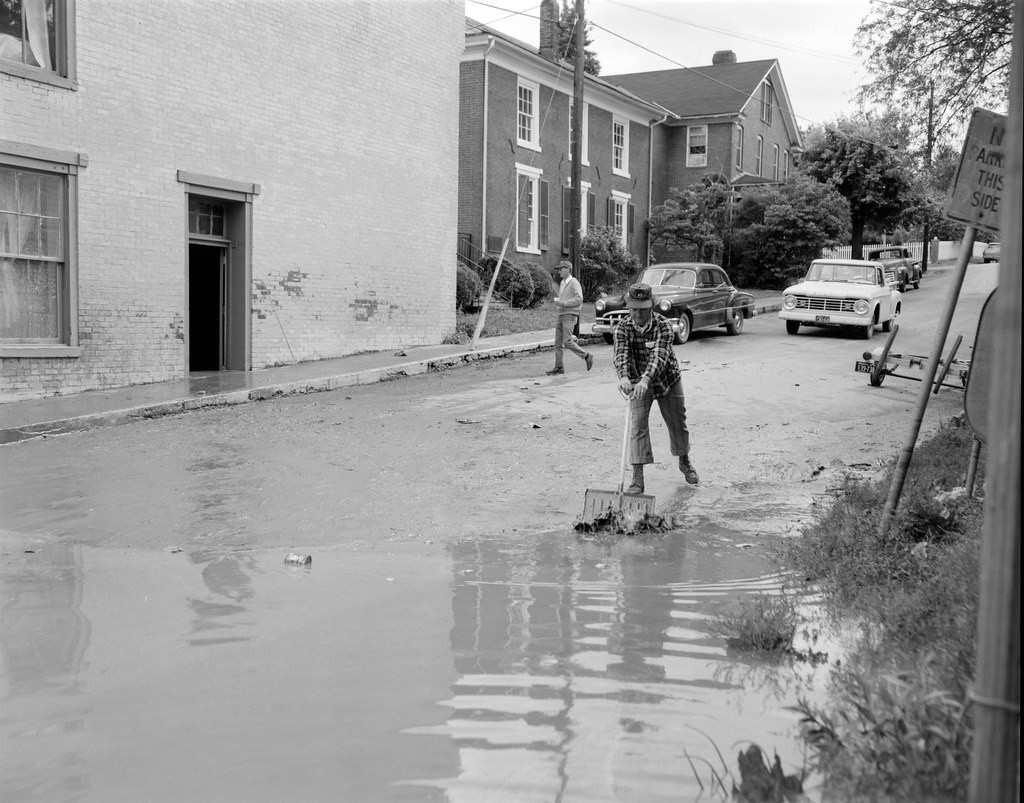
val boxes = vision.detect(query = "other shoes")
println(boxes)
[584,352,593,370]
[545,365,565,375]
[627,480,645,494]
[678,462,700,484]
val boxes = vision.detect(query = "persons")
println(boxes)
[613,284,699,495]
[546,261,594,374]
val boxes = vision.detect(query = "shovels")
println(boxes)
[580,381,657,535]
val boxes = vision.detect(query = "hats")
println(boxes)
[627,283,654,309]
[553,260,574,270]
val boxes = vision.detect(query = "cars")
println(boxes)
[592,262,758,344]
[869,246,922,292]
[779,258,902,338]
[983,242,1000,262]
